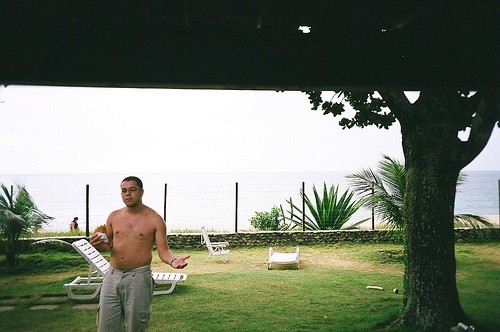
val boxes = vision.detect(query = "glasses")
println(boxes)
[120,187,140,193]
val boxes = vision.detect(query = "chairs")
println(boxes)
[63,239,187,299]
[201,227,230,265]
[268,246,302,269]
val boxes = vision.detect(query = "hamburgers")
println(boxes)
[94,224,107,235]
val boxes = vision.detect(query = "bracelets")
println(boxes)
[171,257,177,267]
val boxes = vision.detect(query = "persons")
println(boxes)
[89,176,190,332]
[71,217,79,231]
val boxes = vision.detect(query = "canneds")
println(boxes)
[469,325,477,331]
[457,322,469,332]
